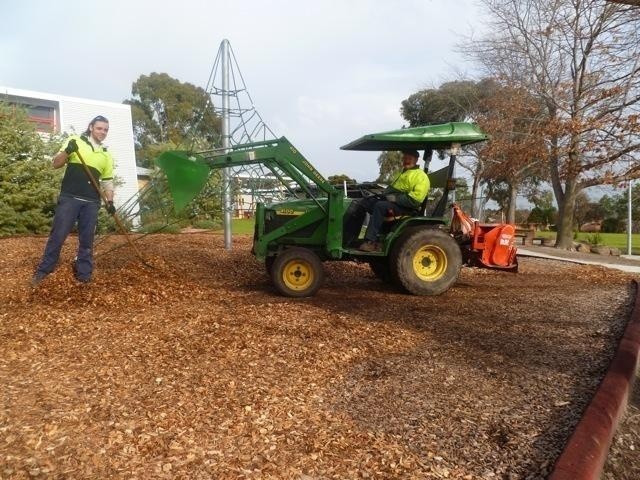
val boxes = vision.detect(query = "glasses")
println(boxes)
[95,116,109,123]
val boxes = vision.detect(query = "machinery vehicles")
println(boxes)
[153,117,520,303]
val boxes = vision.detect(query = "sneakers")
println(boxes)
[359,240,376,252]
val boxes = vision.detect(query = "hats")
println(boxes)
[402,149,419,157]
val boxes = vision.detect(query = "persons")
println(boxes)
[343,147,432,253]
[31,114,115,284]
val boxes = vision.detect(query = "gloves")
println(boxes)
[105,201,116,214]
[386,195,396,202]
[65,139,78,154]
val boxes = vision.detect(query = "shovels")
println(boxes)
[77,150,162,270]
